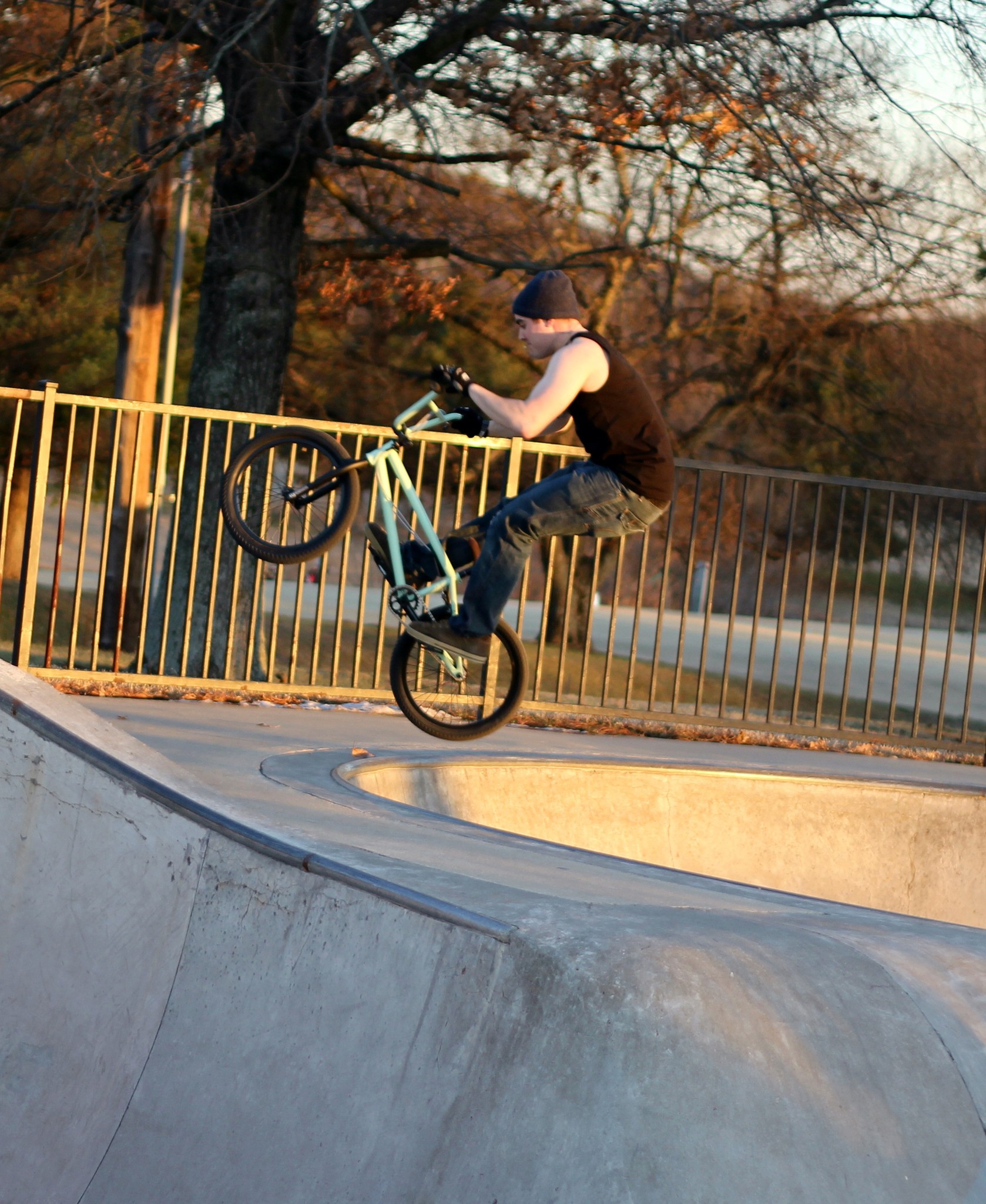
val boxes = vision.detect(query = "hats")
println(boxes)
[511,268,583,323]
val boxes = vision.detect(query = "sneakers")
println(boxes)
[404,617,492,665]
[363,521,424,595]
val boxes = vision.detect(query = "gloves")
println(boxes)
[446,406,489,439]
[419,363,478,398]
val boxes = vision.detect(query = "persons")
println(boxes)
[362,271,677,665]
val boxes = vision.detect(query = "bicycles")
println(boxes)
[215,364,532,742]
